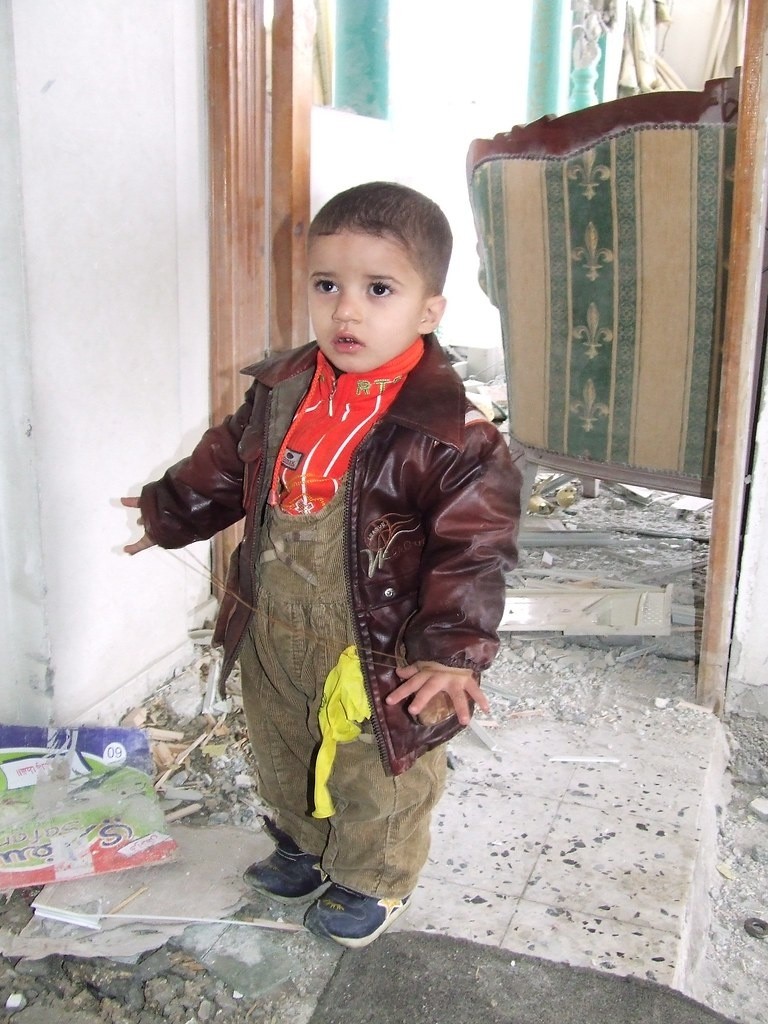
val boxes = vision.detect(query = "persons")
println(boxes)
[122,184,524,950]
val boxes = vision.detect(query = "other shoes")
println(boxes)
[242,814,334,906]
[302,882,413,950]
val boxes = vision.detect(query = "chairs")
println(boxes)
[467,66,741,532]
